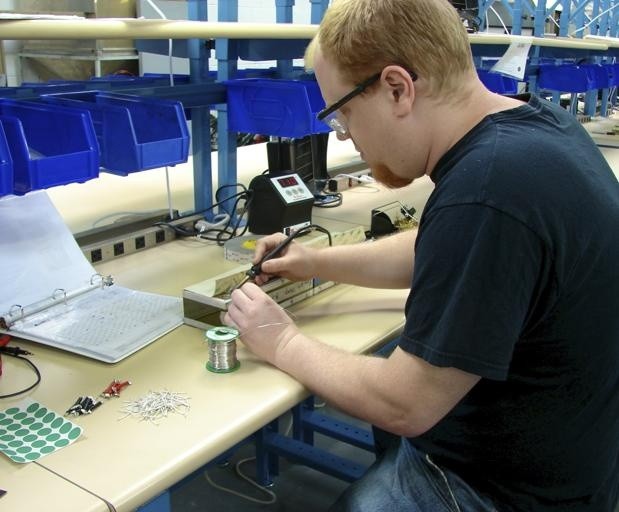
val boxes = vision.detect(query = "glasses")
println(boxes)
[316,72,417,138]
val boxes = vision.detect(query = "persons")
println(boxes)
[219,0,618,511]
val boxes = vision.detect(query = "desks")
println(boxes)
[1,112,619,512]
